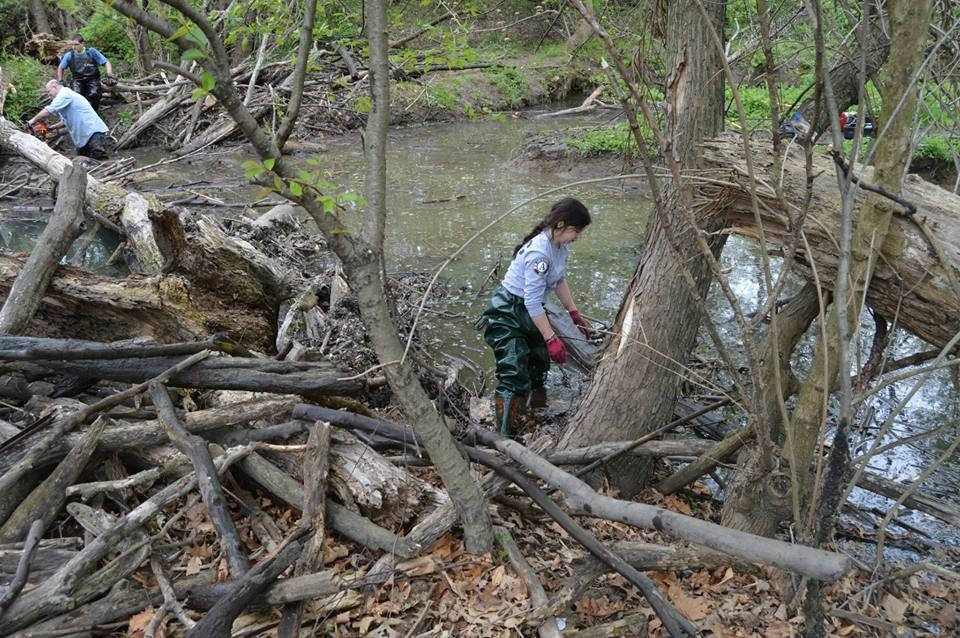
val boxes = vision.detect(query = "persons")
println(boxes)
[57,34,116,115]
[25,79,110,161]
[481,198,591,438]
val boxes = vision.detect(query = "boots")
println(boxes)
[496,396,519,438]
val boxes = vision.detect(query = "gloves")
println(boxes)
[568,309,591,341]
[60,80,66,87]
[22,123,28,131]
[545,334,566,363]
[106,77,113,86]
